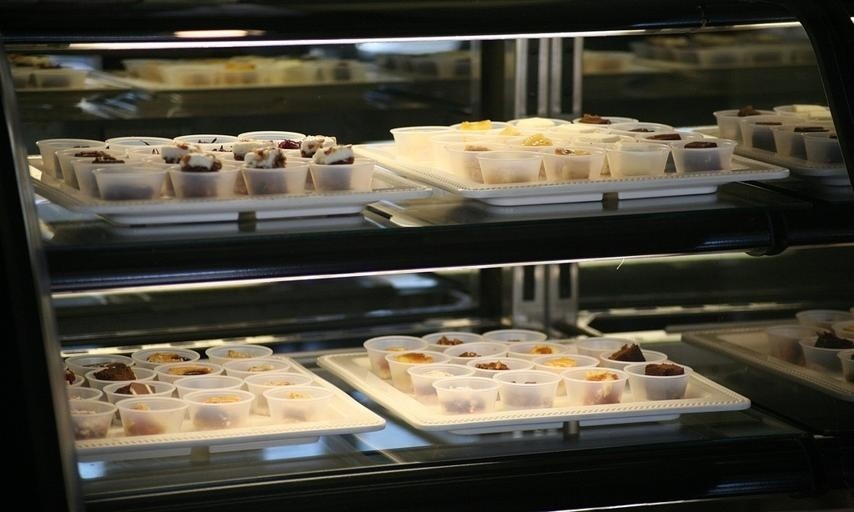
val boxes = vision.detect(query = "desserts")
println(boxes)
[378,335,685,413]
[35,135,355,200]
[408,104,845,178]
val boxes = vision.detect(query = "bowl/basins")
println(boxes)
[63,339,336,437]
[361,325,693,412]
[406,298,781,326]
[765,305,852,377]
[33,129,375,199]
[712,98,840,171]
[7,43,473,114]
[389,112,744,188]
[502,27,822,106]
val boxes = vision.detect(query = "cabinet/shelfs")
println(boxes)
[0,18,852,511]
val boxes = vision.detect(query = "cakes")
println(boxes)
[808,320,853,381]
[651,34,815,65]
[5,54,72,88]
[60,350,312,439]
[137,57,351,85]
